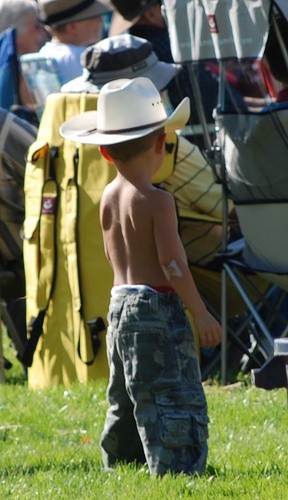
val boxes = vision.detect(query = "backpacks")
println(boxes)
[17,92,119,389]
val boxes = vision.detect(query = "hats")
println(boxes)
[37,0,114,28]
[59,77,190,146]
[60,35,181,94]
[108,1,160,35]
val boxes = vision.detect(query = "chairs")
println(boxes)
[0,0,288,386]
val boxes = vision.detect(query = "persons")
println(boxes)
[0,0,288,384]
[59,33,288,323]
[58,77,224,479]
